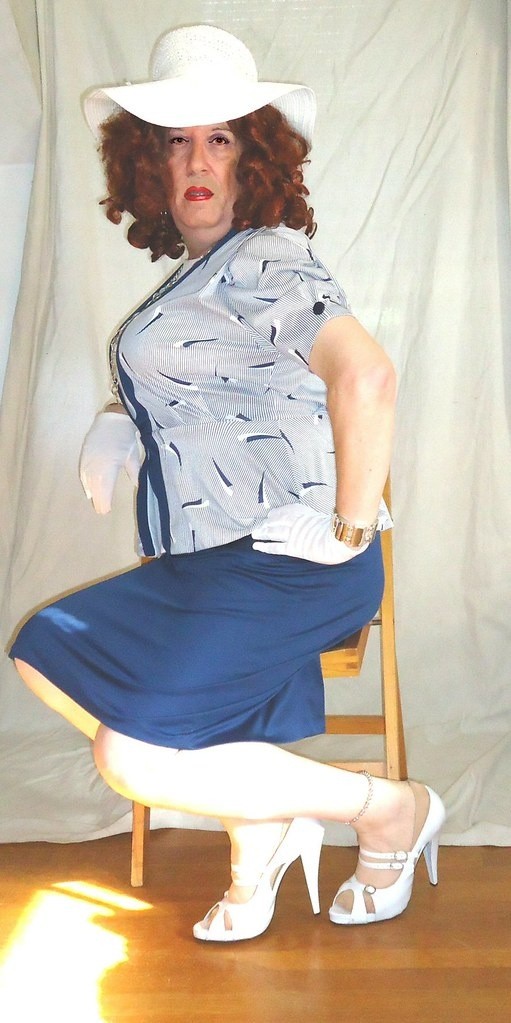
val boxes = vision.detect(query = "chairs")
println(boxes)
[128,464,406,891]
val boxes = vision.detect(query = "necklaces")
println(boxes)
[107,245,210,406]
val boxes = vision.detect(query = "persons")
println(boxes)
[10,25,448,944]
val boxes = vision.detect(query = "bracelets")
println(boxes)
[330,507,379,548]
[103,398,123,410]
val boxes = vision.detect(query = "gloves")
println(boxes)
[252,502,369,564]
[78,410,143,517]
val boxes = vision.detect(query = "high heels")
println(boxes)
[191,815,323,942]
[328,781,445,925]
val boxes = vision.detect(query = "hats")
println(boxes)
[85,25,314,154]
